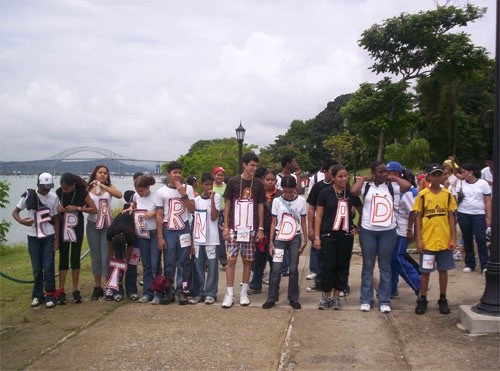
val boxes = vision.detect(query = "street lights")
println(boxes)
[235,120,246,174]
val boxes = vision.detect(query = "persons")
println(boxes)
[12,172,61,308]
[123,171,163,306]
[85,166,122,301]
[306,157,363,310]
[222,153,266,309]
[56,173,97,304]
[189,173,221,304]
[248,154,302,295]
[155,162,195,305]
[262,174,307,310]
[211,167,227,224]
[106,212,140,301]
[187,176,199,196]
[350,153,493,317]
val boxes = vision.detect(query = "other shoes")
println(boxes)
[263,301,275,309]
[415,298,427,314]
[30,292,56,308]
[306,284,321,292]
[380,305,391,312]
[90,286,217,305]
[247,288,261,295]
[339,287,349,296]
[306,272,316,279]
[318,296,331,309]
[438,299,450,314]
[453,251,463,260]
[331,298,340,309]
[463,266,474,271]
[57,291,81,304]
[361,304,371,312]
[289,300,301,309]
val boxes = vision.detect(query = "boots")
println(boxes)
[221,282,250,308]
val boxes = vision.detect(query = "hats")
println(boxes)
[387,162,403,173]
[37,173,54,188]
[429,163,444,174]
[213,167,225,174]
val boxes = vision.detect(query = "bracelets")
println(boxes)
[223,229,229,230]
[309,228,312,230]
[78,207,80,211]
[315,237,319,240]
[259,227,263,230]
[182,195,188,198]
